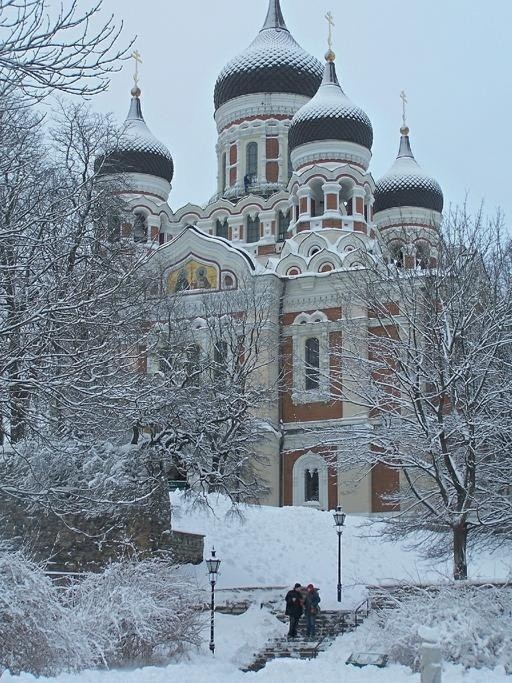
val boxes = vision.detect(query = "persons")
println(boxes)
[284,582,304,639]
[299,583,321,637]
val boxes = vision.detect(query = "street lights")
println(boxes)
[204,542,223,653]
[331,502,347,603]
